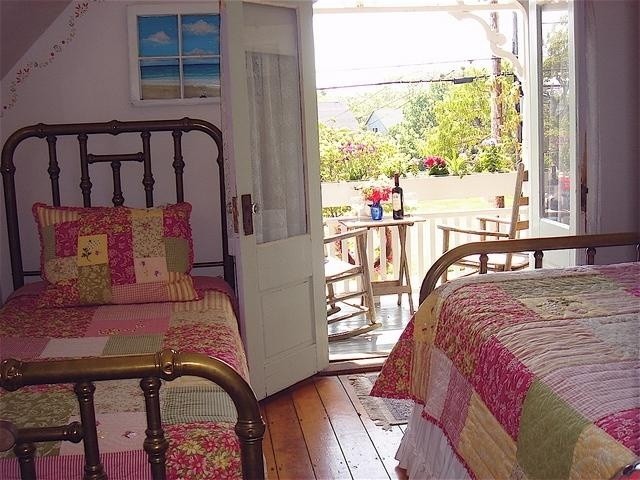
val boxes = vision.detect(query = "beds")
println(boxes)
[368,232,640,480]
[0,118,270,480]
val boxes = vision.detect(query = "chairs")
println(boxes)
[323,224,382,341]
[438,162,528,286]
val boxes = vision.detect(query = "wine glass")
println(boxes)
[350,197,366,222]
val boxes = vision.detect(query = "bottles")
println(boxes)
[392,174,403,220]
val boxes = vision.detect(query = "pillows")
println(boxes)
[31,203,204,309]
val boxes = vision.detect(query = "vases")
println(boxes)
[371,202,382,220]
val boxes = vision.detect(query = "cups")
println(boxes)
[495,196,504,208]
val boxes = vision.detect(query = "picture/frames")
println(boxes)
[127,5,223,107]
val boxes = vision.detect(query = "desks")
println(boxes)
[337,214,426,315]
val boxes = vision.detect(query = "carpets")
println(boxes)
[347,371,414,431]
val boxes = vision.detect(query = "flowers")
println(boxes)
[361,184,392,207]
[423,156,448,175]
